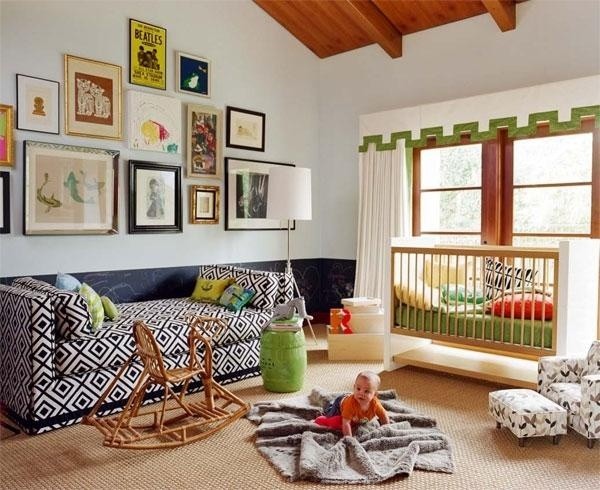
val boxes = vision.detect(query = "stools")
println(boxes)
[260,328,306,393]
[487,387,568,447]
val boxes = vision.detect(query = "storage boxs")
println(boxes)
[326,310,384,361]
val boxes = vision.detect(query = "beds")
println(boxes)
[382,236,600,393]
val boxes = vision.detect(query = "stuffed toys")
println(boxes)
[261,295,307,332]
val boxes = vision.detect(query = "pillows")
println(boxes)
[12,273,117,339]
[393,260,552,319]
[192,264,278,310]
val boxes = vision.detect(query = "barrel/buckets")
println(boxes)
[260,315,308,392]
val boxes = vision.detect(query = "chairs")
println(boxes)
[537,340,600,449]
[80,317,251,450]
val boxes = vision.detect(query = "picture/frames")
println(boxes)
[225,156,296,230]
[129,18,167,91]
[176,52,211,98]
[226,106,266,153]
[186,103,223,180]
[128,159,184,233]
[190,184,219,225]
[0,55,122,236]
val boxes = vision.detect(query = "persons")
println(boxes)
[148,179,166,218]
[192,111,216,169]
[138,46,160,72]
[323,370,389,436]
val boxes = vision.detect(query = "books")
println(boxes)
[270,313,305,332]
[341,297,382,314]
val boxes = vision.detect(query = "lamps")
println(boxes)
[266,168,319,345]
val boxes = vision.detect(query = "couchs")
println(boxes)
[0,271,294,435]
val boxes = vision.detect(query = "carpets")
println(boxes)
[245,388,454,484]
